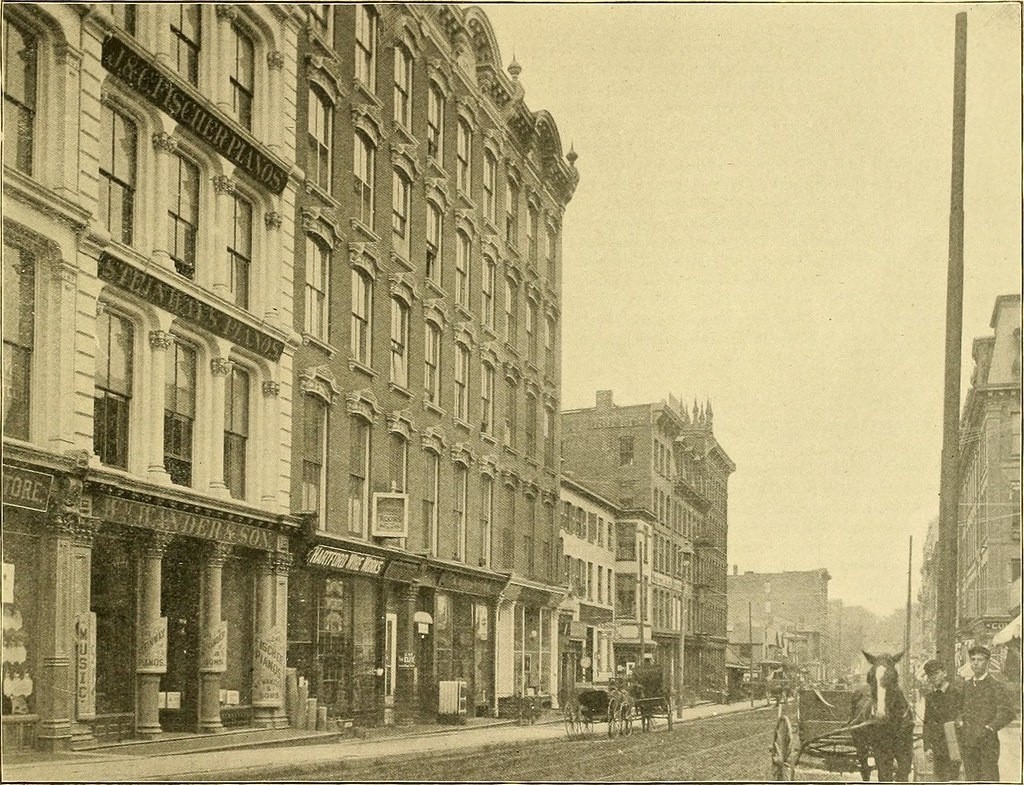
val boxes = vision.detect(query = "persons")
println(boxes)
[918,642,1015,782]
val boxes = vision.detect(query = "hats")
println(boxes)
[967,646,991,658]
[920,659,943,678]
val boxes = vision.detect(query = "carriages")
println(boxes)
[769,649,915,782]
[564,664,673,738]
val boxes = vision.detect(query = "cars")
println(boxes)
[741,670,817,697]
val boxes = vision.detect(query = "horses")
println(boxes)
[848,649,917,782]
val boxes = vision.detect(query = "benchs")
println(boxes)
[799,690,856,741]
[578,691,608,708]
